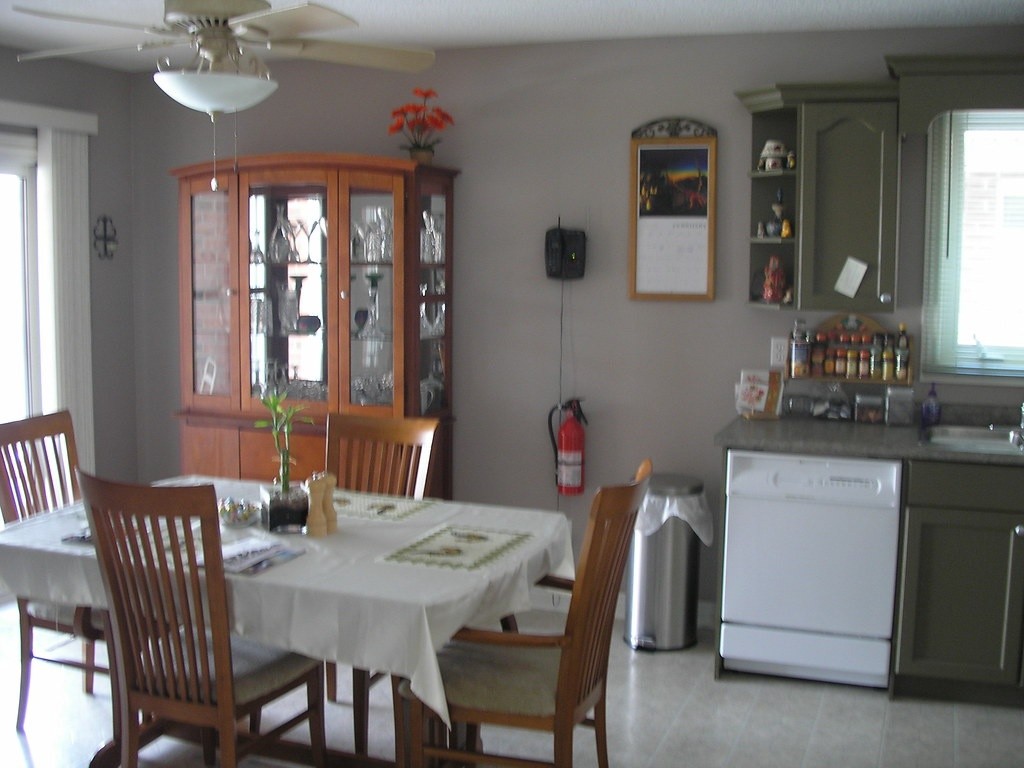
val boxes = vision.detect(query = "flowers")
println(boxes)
[386,86,455,149]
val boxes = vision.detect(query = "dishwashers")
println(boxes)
[719,448,902,686]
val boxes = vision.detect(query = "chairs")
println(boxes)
[1,409,156,736]
[72,463,328,768]
[397,454,654,768]
[325,410,446,703]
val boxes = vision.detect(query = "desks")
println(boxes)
[0,473,577,768]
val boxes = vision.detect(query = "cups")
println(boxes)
[354,378,392,406]
[278,290,297,331]
[420,383,433,415]
[250,299,273,334]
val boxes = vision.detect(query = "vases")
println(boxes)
[410,149,434,165]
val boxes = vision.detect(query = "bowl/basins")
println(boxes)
[298,316,320,334]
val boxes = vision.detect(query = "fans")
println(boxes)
[10,0,437,76]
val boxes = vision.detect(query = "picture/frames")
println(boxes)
[627,134,716,304]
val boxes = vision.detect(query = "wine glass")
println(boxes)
[254,357,301,401]
[350,224,392,262]
[424,211,443,264]
[248,217,327,265]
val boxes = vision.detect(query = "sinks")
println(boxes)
[927,429,1014,447]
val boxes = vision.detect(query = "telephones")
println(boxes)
[543,217,587,278]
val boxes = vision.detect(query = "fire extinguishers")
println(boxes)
[548,398,590,495]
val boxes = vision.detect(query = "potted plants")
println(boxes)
[253,384,316,537]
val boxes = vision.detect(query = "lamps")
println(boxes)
[153,26,279,125]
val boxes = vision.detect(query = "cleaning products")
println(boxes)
[920,383,941,428]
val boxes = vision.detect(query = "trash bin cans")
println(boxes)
[620,472,702,652]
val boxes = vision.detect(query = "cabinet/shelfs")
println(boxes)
[161,151,461,502]
[730,82,896,314]
[888,458,1024,707]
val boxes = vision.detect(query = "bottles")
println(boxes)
[271,205,288,262]
[420,284,444,337]
[921,379,942,425]
[790,317,909,380]
